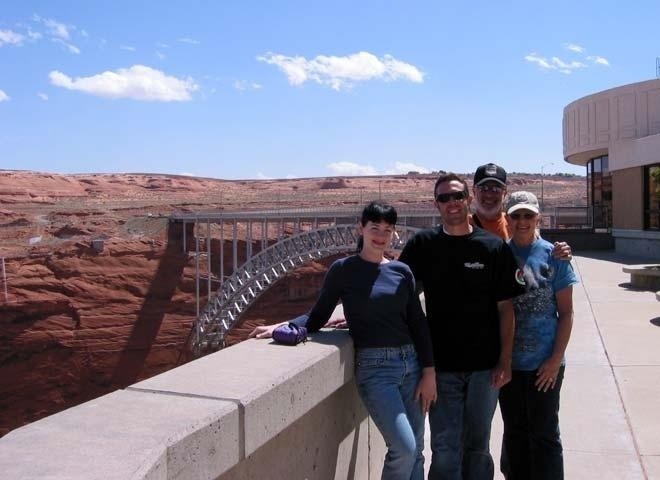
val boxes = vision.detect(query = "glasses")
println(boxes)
[436,190,467,203]
[510,210,536,219]
[475,184,503,193]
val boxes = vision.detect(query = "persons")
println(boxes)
[398,173,528,480]
[391,224,403,249]
[468,162,573,262]
[248,200,439,479]
[498,191,578,480]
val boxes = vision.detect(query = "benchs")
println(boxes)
[623,264,660,290]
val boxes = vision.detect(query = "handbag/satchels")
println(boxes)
[272,322,308,346]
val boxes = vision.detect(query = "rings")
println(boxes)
[548,377,553,382]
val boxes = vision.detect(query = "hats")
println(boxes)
[474,163,507,187]
[505,190,539,215]
